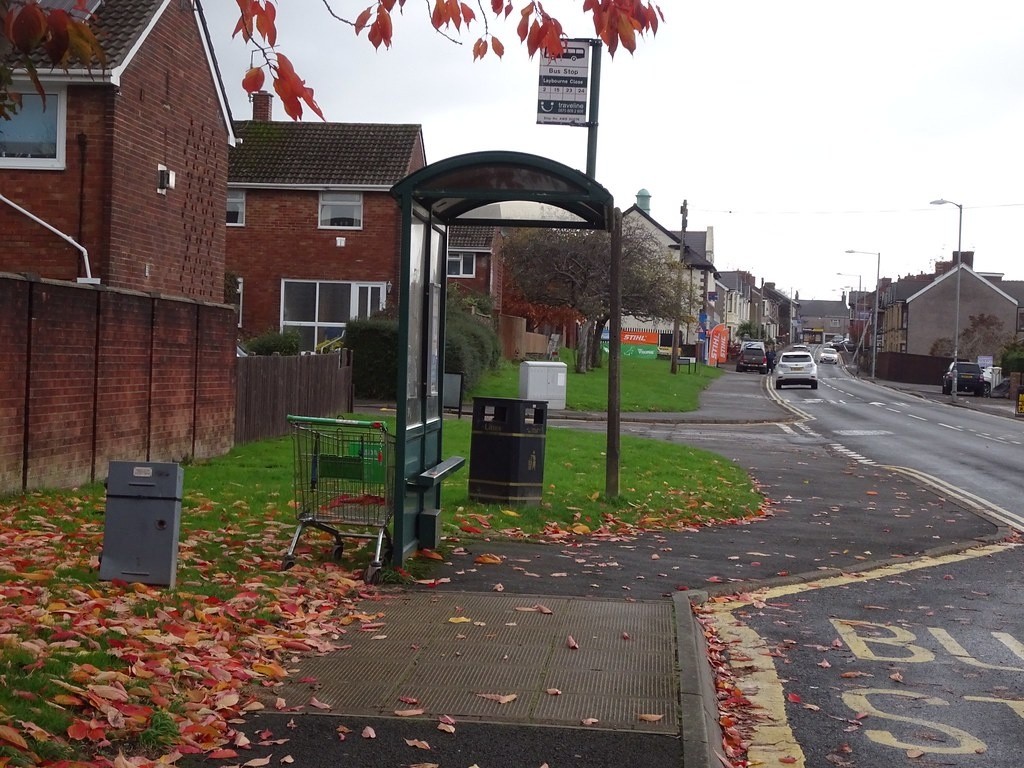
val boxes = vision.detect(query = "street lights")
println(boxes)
[929,199,963,403]
[837,273,861,344]
[833,289,847,340]
[844,250,881,380]
[844,286,855,343]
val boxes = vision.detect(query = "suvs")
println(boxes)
[735,347,767,374]
[941,362,984,397]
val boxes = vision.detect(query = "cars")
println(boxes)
[981,369,992,398]
[773,351,820,390]
[820,347,840,364]
[236,339,256,357]
[789,345,811,352]
[831,335,843,343]
[830,341,856,352]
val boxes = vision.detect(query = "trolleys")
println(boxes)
[281,414,396,586]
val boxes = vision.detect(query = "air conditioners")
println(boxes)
[160,170,175,190]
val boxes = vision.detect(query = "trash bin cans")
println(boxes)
[467,395,550,507]
